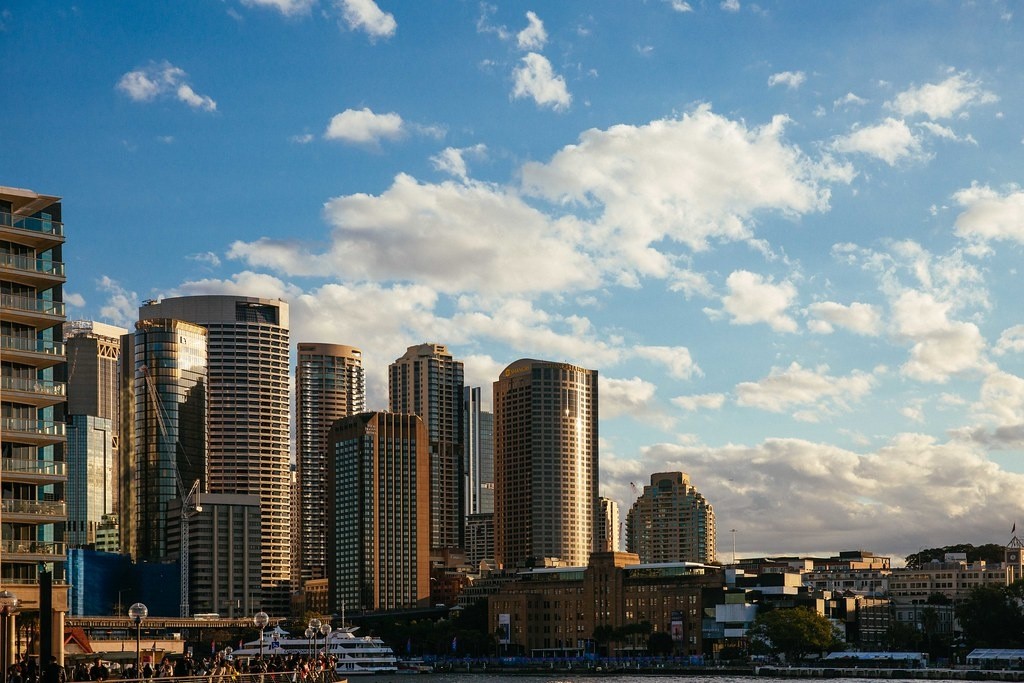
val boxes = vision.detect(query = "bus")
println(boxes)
[194,614,219,621]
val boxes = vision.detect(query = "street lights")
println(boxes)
[128,602,148,679]
[0,589,18,683]
[273,633,278,656]
[254,611,269,660]
[305,618,332,660]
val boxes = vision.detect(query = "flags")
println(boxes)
[1011,523,1015,534]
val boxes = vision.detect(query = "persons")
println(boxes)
[249,652,284,683]
[286,652,339,683]
[89,657,109,683]
[7,654,88,683]
[122,653,245,683]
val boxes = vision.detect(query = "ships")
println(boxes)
[228,597,398,676]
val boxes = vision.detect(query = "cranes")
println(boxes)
[141,365,203,618]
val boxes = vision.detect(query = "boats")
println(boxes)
[396,660,434,675]
